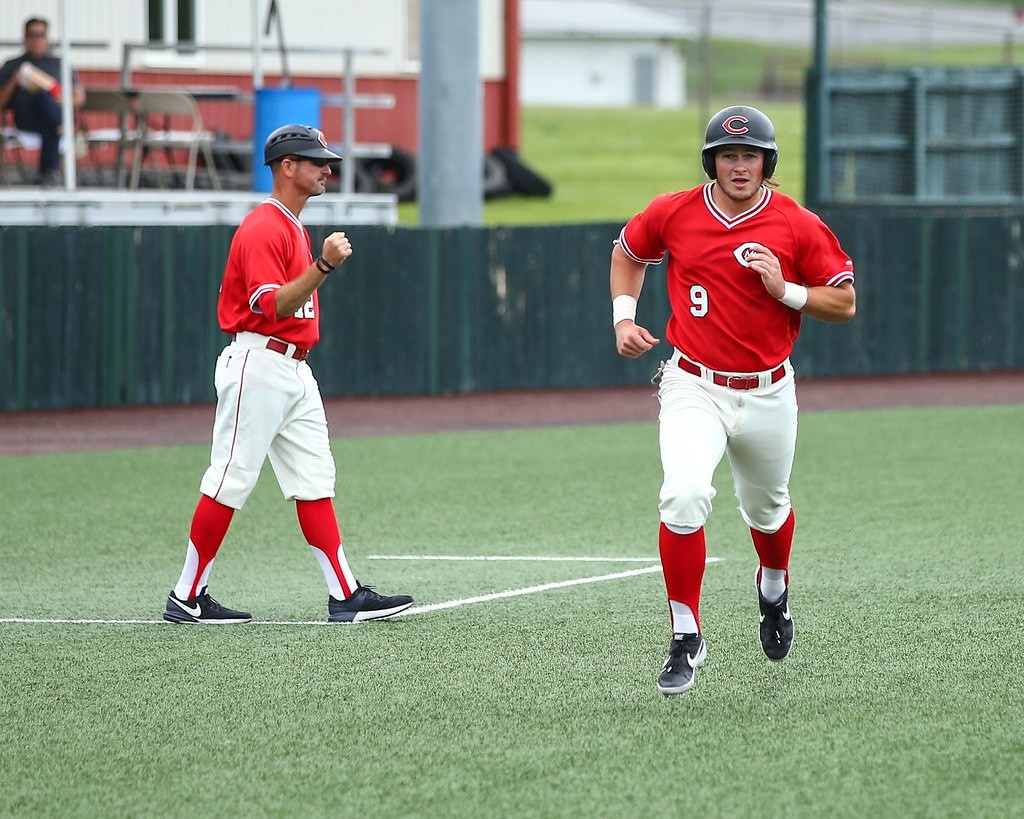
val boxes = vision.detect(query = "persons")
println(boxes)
[610,106,855,693]
[163,124,414,624]
[0,19,87,186]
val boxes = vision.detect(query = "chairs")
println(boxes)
[0,86,224,192]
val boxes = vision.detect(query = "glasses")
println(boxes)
[278,158,329,167]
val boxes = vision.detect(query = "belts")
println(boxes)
[678,357,785,390]
[232,332,309,362]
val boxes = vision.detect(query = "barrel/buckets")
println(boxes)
[251,86,319,193]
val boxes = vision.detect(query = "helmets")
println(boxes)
[701,105,778,180]
[264,125,342,167]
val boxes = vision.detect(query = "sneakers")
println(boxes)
[163,585,252,625]
[657,632,707,696]
[327,580,413,621]
[754,565,795,661]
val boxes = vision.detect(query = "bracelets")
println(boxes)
[316,257,335,274]
[613,295,637,328]
[778,281,807,310]
